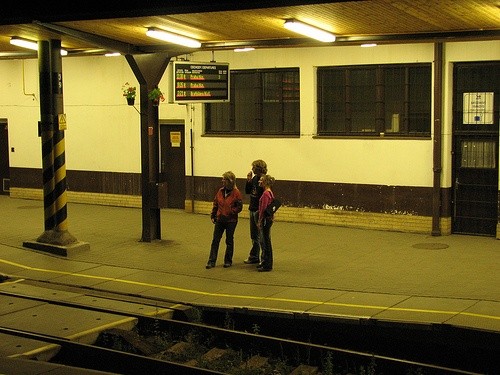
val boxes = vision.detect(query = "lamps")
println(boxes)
[146,27,201,49]
[9,36,68,56]
[283,18,336,43]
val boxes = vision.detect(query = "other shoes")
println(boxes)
[206,263,215,269]
[243,258,260,264]
[224,264,230,268]
[255,262,271,272]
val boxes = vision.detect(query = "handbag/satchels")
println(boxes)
[265,191,282,216]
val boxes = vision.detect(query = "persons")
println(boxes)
[256,175,275,272]
[243,160,268,264]
[205,171,243,269]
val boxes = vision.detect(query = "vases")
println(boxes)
[152,99,159,106]
[127,98,135,105]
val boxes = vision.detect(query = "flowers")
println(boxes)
[147,88,165,102]
[121,82,136,100]
